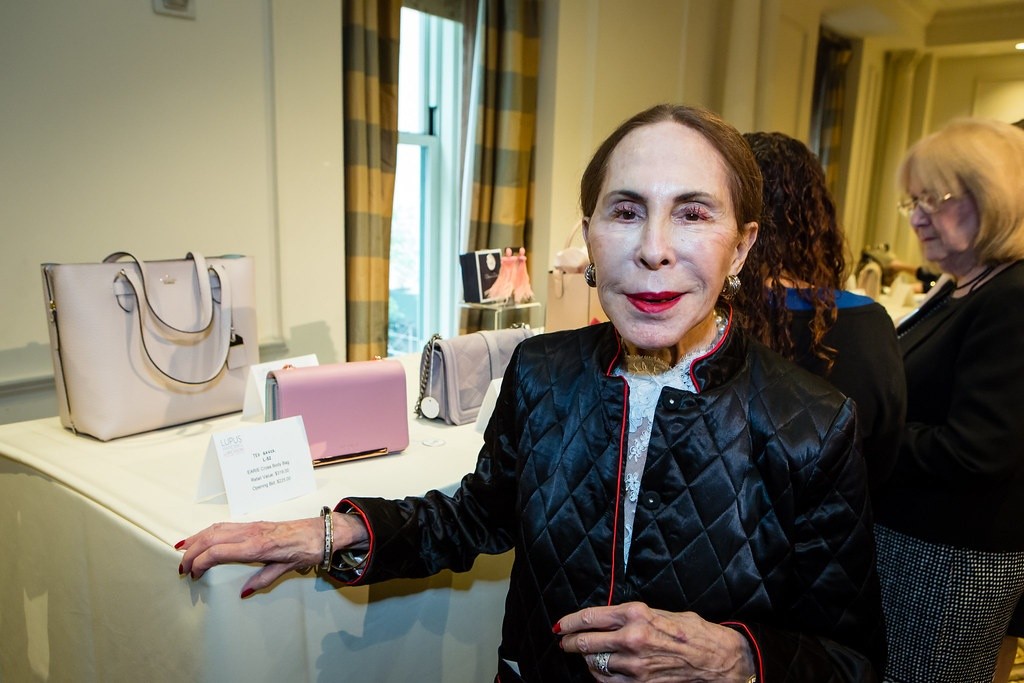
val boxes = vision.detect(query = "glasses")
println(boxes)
[896,187,971,217]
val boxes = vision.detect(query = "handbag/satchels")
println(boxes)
[859,262,884,301]
[38,252,256,443]
[414,322,532,426]
[265,357,409,468]
[856,242,900,287]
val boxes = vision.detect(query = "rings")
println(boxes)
[593,651,615,678]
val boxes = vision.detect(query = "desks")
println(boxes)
[0,353,515,683]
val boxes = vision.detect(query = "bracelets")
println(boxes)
[319,503,333,571]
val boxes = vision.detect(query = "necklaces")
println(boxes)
[712,306,728,354]
[894,256,1008,362]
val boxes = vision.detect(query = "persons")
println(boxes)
[481,244,534,307]
[848,237,943,329]
[877,115,1024,683]
[172,103,889,683]
[720,130,906,546]
